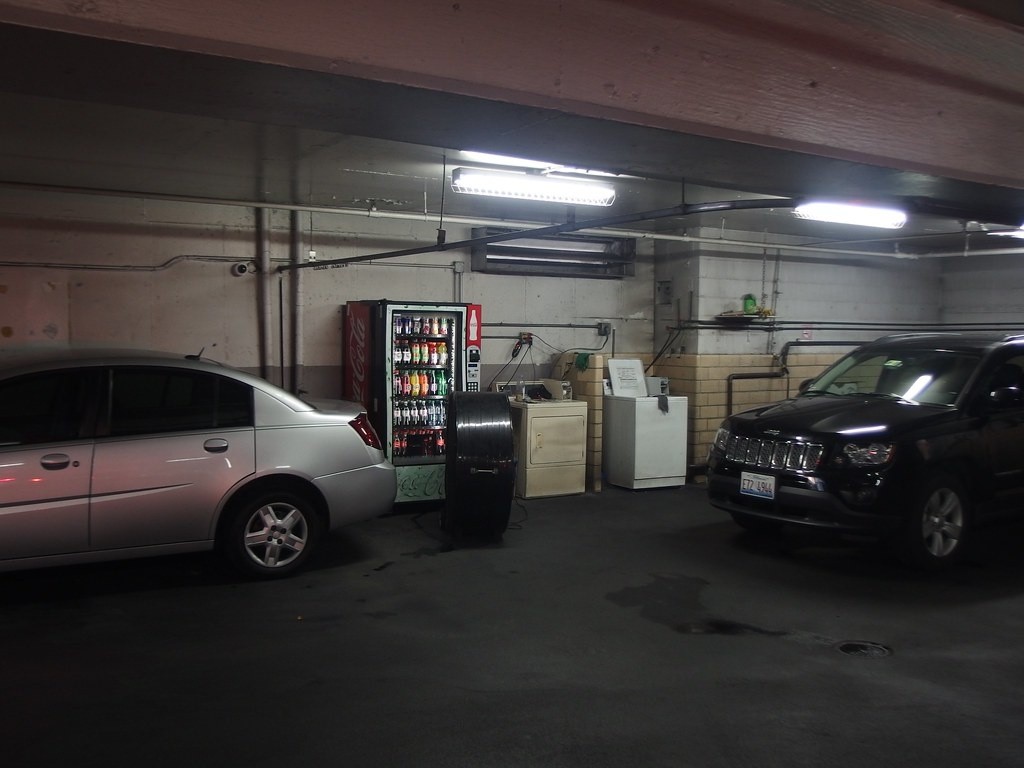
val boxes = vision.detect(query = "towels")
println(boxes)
[658,395,668,416]
[575,353,590,373]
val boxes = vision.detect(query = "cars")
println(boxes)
[1,345,399,586]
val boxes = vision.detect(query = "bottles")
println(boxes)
[393,317,448,457]
[516,376,526,402]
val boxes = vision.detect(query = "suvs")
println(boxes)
[706,330,1024,572]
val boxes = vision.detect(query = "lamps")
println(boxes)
[450,167,616,207]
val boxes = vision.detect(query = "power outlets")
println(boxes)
[598,323,611,336]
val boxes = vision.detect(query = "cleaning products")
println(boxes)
[744,294,756,313]
